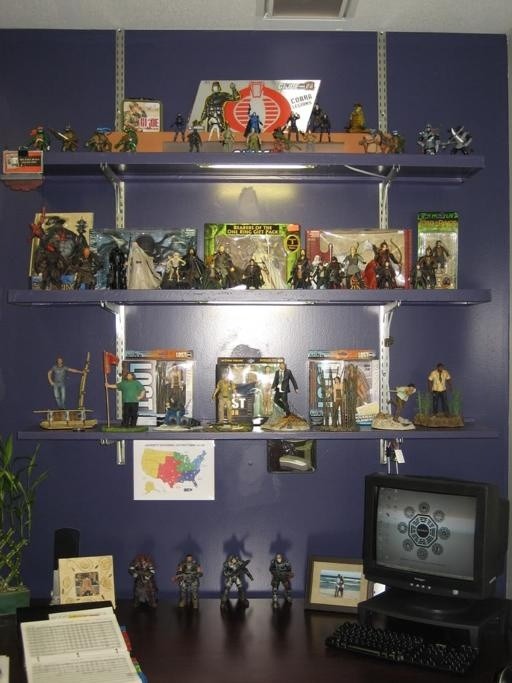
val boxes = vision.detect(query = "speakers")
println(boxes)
[51,528,79,605]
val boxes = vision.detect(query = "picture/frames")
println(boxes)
[301,552,374,614]
[55,551,118,608]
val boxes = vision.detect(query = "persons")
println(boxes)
[269,550,294,610]
[127,553,160,607]
[29,80,453,426]
[218,551,254,611]
[172,552,204,610]
[334,573,340,597]
[338,576,344,597]
[80,573,96,596]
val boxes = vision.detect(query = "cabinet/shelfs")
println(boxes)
[4,141,498,443]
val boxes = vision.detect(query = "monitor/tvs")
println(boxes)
[361,471,506,611]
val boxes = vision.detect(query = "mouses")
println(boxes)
[497,663,512,683]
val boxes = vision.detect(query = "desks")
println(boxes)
[1,590,511,682]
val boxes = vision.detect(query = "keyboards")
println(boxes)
[323,621,477,675]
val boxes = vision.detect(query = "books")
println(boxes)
[18,606,148,682]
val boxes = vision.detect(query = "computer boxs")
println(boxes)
[358,594,506,649]
